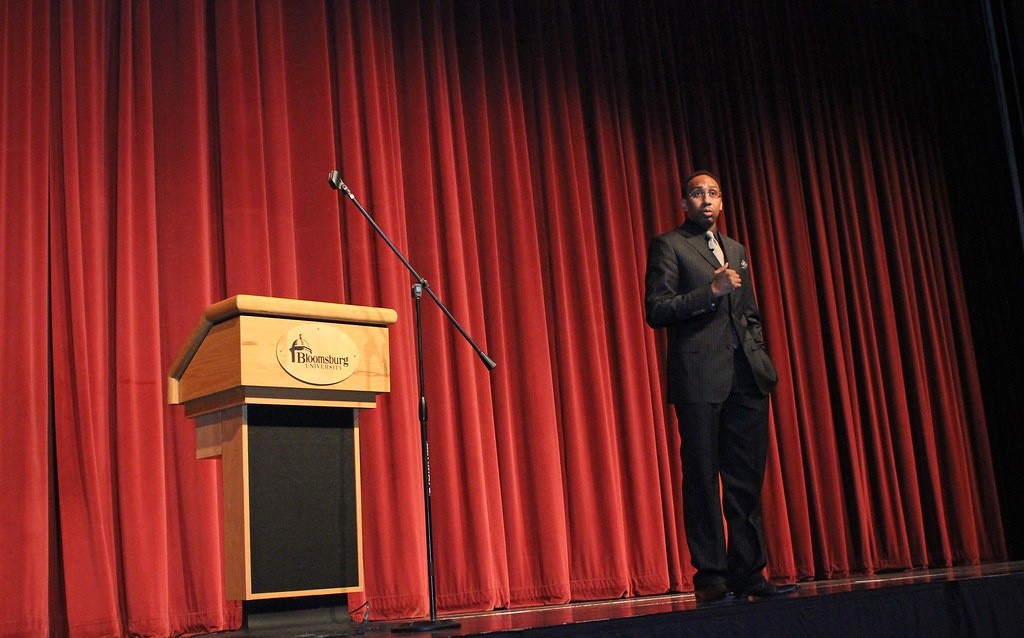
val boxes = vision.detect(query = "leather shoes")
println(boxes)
[727,572,800,599]
[694,578,737,609]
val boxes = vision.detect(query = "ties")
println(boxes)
[706,231,725,267]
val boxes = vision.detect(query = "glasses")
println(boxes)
[686,188,721,201]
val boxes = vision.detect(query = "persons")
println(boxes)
[642,169,805,607]
